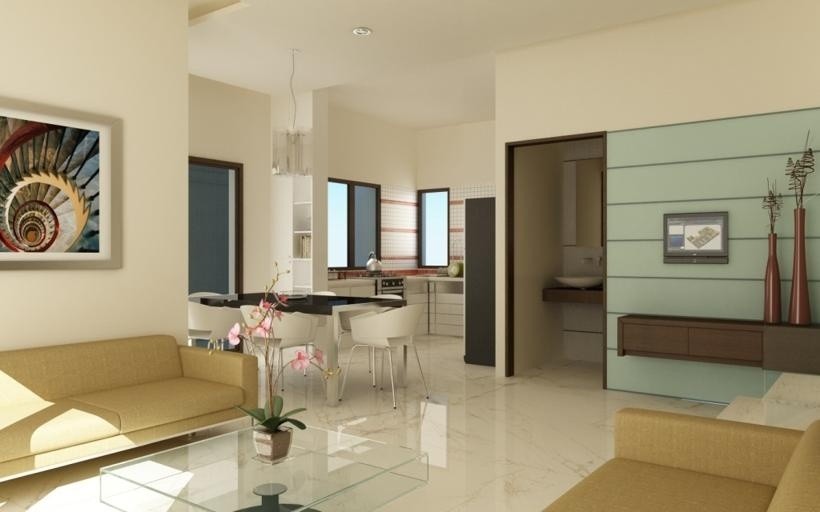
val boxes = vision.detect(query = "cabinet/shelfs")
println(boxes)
[270,176,328,295]
[617,312,820,375]
[429,277,465,338]
[325,277,377,298]
[405,277,429,339]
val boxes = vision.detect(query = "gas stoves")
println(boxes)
[347,272,403,286]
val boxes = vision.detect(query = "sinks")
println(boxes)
[555,275,603,288]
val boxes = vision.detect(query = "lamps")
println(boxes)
[270,50,312,176]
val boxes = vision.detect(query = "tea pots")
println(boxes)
[366,251,382,271]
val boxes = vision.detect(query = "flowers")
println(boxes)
[206,261,342,429]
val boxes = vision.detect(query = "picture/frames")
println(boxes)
[0,97,123,271]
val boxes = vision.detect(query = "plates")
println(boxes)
[448,262,460,277]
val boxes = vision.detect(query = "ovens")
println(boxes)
[376,287,406,308]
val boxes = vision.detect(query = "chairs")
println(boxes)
[339,303,429,409]
[188,296,240,347]
[189,291,220,307]
[338,293,404,389]
[306,292,336,296]
[539,406,820,512]
[238,304,322,395]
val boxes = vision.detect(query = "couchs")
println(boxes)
[0,333,259,485]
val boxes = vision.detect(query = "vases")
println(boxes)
[763,231,782,324]
[251,430,292,465]
[785,206,815,326]
